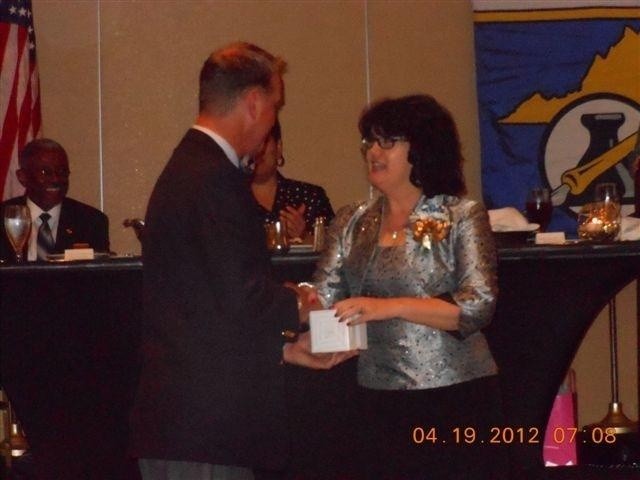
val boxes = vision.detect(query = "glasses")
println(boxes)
[360,134,407,150]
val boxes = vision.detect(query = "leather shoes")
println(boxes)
[37,213,55,262]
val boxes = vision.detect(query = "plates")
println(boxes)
[288,245,315,254]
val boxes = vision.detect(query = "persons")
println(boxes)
[245,112,335,246]
[296,93,507,478]
[0,138,111,262]
[133,42,359,479]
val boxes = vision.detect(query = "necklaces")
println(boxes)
[384,207,410,242]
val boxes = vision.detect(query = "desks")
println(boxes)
[0,241,640,469]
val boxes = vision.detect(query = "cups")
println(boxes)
[596,183,623,222]
[526,188,553,232]
[266,220,292,256]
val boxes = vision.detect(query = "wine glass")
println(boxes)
[4,204,33,262]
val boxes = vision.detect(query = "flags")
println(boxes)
[0,0,42,203]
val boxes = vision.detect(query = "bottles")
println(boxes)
[564,111,634,205]
[313,215,330,254]
